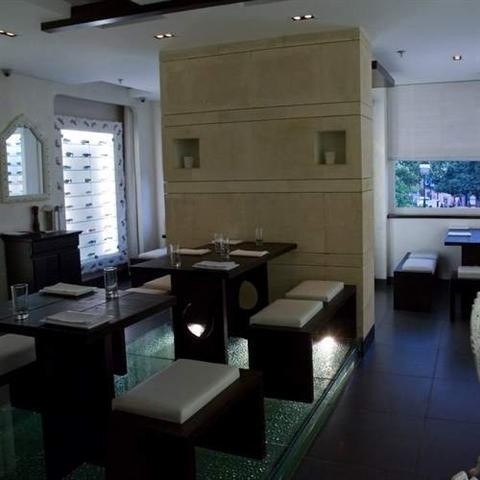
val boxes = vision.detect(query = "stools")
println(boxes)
[0,331,40,415]
[453,266,479,318]
[112,358,269,479]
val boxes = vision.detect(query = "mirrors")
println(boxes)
[0,112,51,203]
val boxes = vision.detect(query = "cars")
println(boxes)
[417,197,451,208]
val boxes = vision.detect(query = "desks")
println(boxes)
[127,238,295,362]
[442,227,479,309]
[0,280,175,479]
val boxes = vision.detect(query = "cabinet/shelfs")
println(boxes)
[1,231,82,299]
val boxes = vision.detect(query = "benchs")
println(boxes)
[251,282,357,404]
[391,247,441,308]
[127,271,172,293]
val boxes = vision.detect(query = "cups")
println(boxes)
[167,240,181,267]
[214,232,230,261]
[10,283,29,320]
[183,156,193,169]
[324,151,337,165]
[254,222,263,246]
[103,267,120,300]
